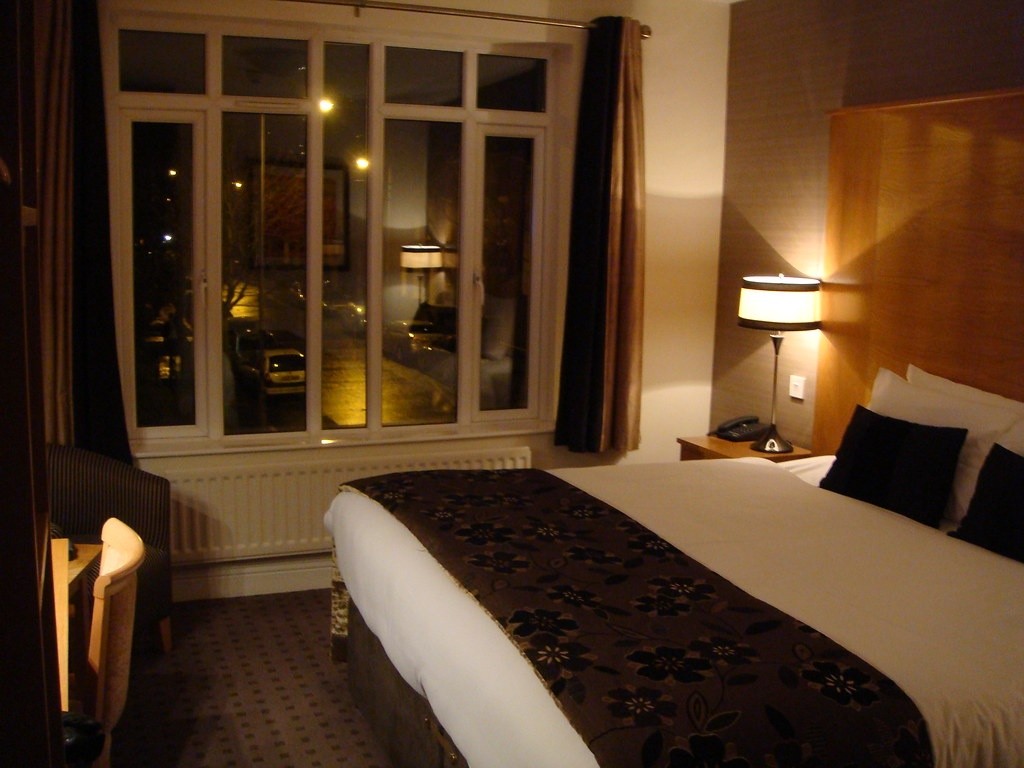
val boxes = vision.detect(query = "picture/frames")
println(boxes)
[246,158,352,273]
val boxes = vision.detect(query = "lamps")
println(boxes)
[401,245,443,306]
[737,274,821,453]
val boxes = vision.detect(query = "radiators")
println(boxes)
[163,445,570,567]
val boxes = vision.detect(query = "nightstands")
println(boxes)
[674,436,811,462]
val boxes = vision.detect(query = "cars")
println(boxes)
[240,328,307,402]
[293,274,368,336]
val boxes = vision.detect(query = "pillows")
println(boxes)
[482,297,515,358]
[945,442,1024,563]
[997,417,1024,457]
[815,402,969,529]
[906,363,1024,415]
[866,367,1021,524]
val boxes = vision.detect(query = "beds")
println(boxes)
[322,453,1024,768]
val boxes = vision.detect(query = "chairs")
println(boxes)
[43,442,175,652]
[70,515,146,768]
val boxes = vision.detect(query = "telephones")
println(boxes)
[715,416,769,443]
